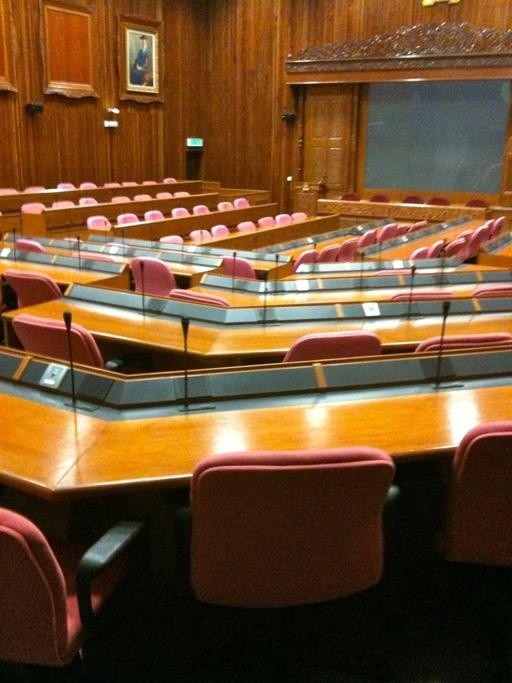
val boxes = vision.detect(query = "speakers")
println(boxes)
[26,104,43,113]
[282,113,295,121]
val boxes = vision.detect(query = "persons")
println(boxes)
[131,35,152,86]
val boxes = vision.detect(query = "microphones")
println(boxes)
[435,238,448,288]
[401,265,426,321]
[308,242,321,274]
[63,311,99,411]
[180,317,216,411]
[270,254,286,295]
[11,218,211,271]
[137,262,160,317]
[356,252,371,291]
[431,301,463,389]
[229,252,246,295]
[370,239,387,271]
[254,220,389,255]
[257,272,280,326]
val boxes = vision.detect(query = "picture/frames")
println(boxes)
[115,12,167,105]
